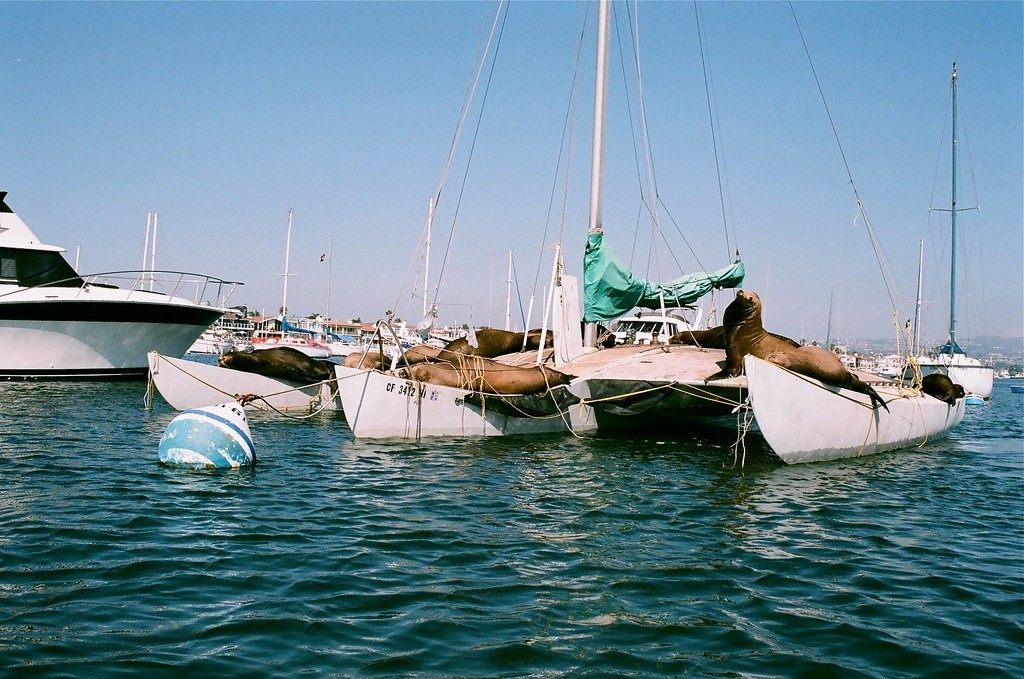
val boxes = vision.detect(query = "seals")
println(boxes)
[668,290,891,415]
[214,321,616,395]
[914,373,965,407]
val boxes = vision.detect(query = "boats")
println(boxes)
[964,395,984,408]
[191,2,965,466]
[1011,385,1024,393]
[144,350,343,414]
[0,186,245,378]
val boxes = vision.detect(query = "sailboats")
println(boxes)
[900,60,994,402]
